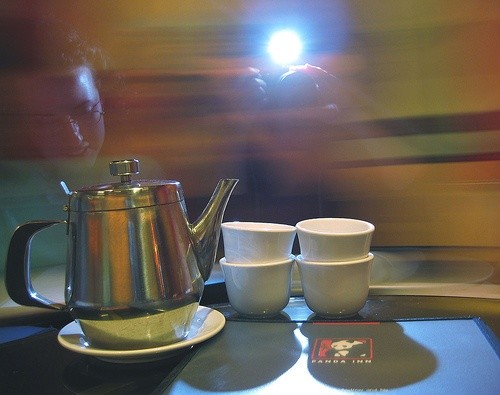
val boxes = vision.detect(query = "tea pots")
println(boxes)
[4,159,239,351]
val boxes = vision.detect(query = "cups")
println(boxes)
[218,253,296,319]
[296,252,375,319]
[296,217,375,263]
[221,221,295,265]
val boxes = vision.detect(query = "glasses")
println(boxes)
[32,94,104,136]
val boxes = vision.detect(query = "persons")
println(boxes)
[209,63,375,256]
[0,14,169,270]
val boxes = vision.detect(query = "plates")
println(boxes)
[57,304,225,362]
